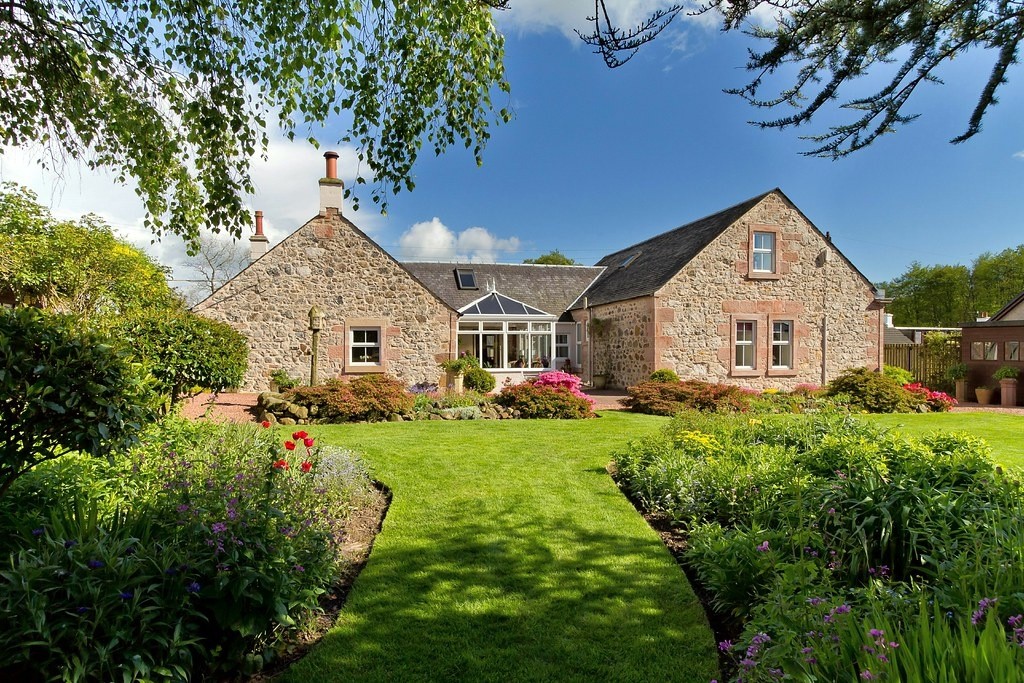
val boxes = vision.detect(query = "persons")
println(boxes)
[539,356,549,368]
[515,355,526,368]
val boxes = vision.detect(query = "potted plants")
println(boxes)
[593,374,607,390]
[975,385,994,405]
[992,366,1021,406]
[945,362,971,402]
